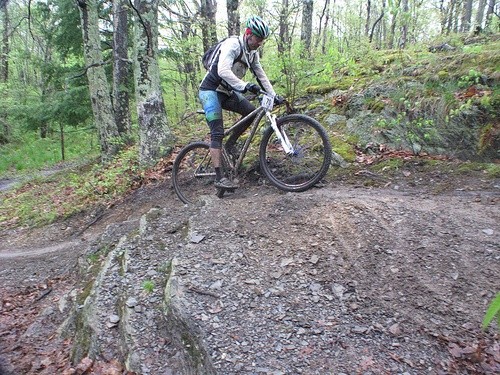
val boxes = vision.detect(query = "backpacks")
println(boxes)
[202,37,256,70]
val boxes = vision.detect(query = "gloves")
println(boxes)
[245,83,260,94]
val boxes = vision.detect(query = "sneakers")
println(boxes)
[224,144,240,158]
[214,178,238,188]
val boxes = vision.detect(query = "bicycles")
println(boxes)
[171,83,332,205]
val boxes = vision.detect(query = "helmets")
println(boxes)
[246,16,270,39]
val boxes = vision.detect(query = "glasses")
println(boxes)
[254,36,262,43]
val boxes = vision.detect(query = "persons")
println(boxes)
[198,16,285,190]
